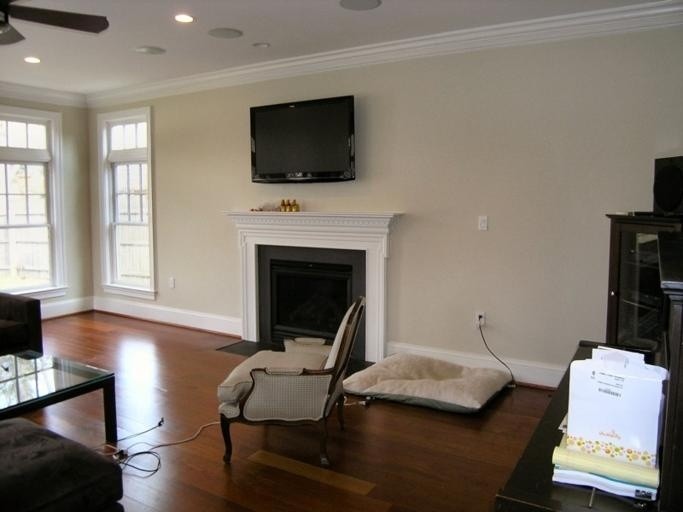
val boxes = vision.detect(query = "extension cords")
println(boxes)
[364,395,371,408]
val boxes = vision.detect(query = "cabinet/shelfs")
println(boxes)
[605,213,682,348]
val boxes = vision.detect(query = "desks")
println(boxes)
[495,339,664,512]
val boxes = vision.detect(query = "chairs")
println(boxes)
[218,294,365,465]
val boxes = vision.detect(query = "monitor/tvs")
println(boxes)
[249,95,356,184]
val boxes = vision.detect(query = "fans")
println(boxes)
[0,0,108,44]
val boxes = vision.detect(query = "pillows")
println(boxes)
[344,350,512,415]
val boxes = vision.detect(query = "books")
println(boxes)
[550,345,669,500]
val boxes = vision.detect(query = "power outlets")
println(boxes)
[474,312,488,324]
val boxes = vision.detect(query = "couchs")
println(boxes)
[1,294,43,354]
[1,418,123,511]
